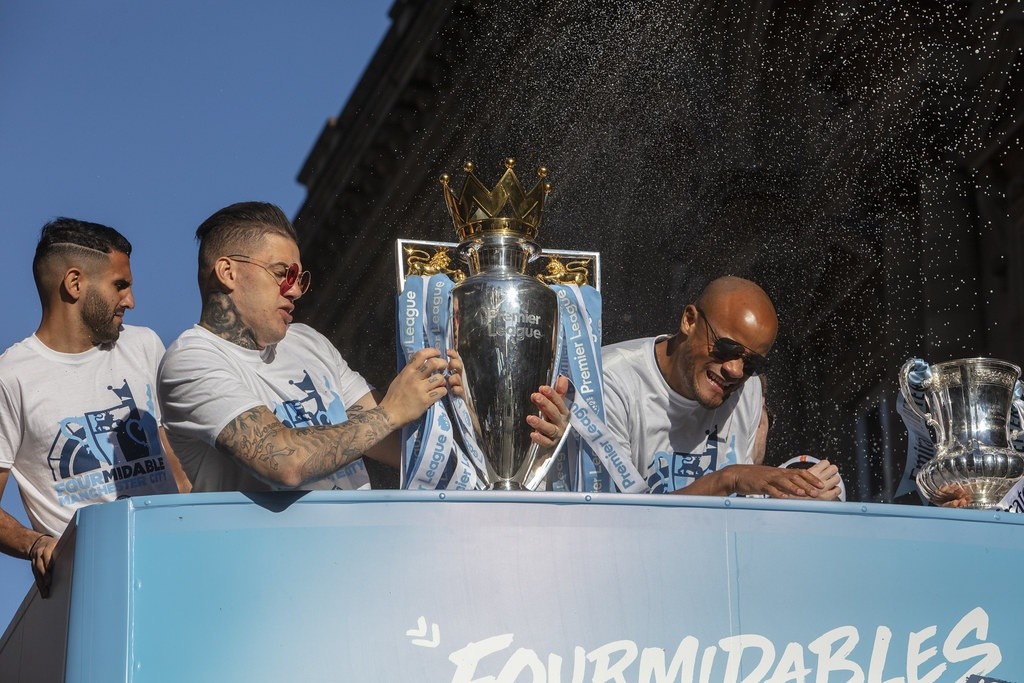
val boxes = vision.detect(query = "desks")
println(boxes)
[0,489,1024,683]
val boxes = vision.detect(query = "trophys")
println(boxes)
[440,158,561,491]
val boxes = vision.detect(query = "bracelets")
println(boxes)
[28,533,54,560]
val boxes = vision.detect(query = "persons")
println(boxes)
[601,276,825,499]
[753,374,842,500]
[156,200,571,493]
[942,497,967,509]
[453,294,483,438]
[0,217,193,600]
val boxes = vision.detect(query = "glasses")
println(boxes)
[696,307,771,376]
[211,253,311,294]
